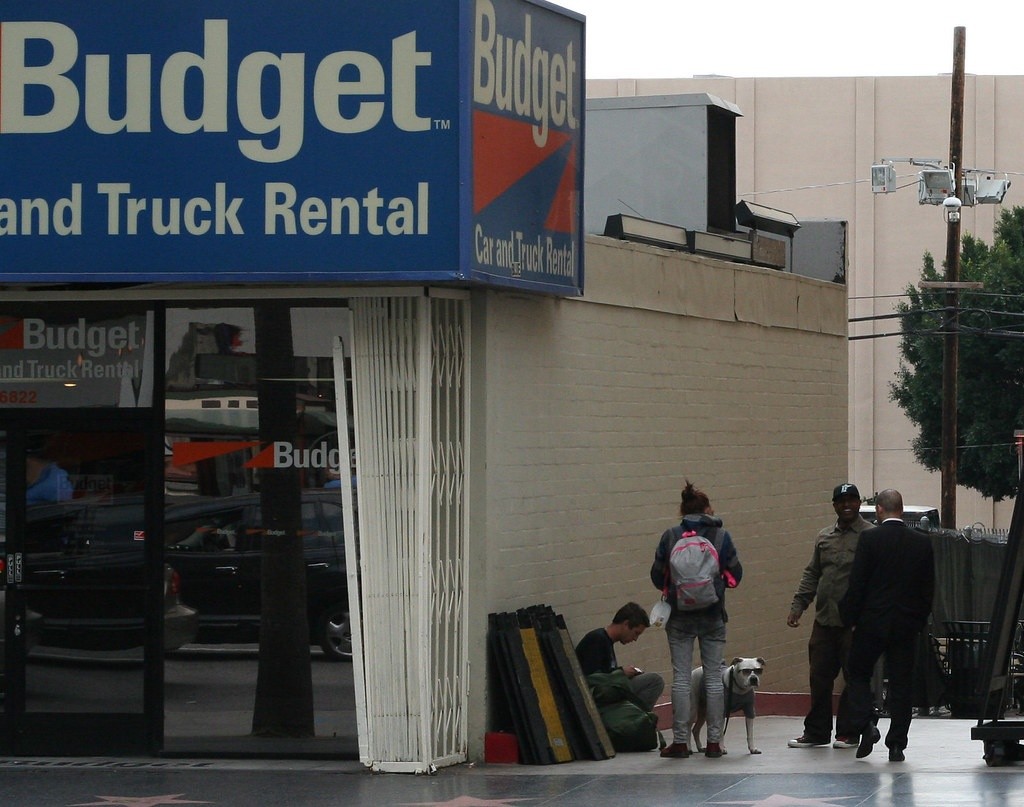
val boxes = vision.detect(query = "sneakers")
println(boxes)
[833,735,860,748]
[788,731,832,748]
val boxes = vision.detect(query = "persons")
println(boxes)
[650,478,743,759]
[785,483,879,748]
[837,489,936,764]
[573,601,667,754]
[27,429,86,506]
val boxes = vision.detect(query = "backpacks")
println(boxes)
[587,669,667,752]
[669,525,725,611]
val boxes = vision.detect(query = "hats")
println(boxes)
[832,484,859,501]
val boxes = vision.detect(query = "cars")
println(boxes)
[0,484,365,664]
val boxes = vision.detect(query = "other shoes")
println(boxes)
[660,743,689,758]
[705,739,722,757]
[856,729,881,758]
[889,748,905,761]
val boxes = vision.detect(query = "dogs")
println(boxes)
[687,657,766,754]
